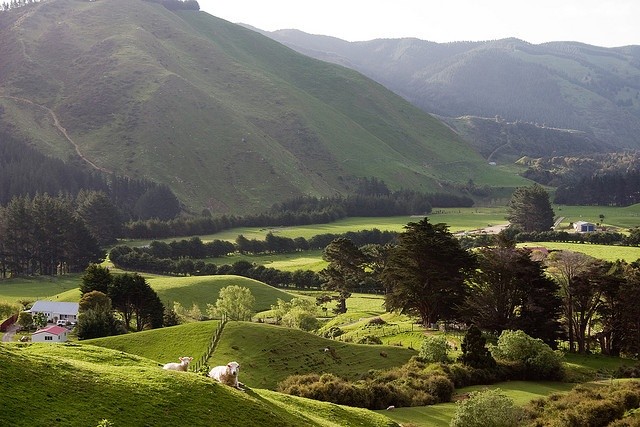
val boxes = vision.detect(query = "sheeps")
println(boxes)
[162,357,194,371]
[208,362,246,393]
[387,405,395,411]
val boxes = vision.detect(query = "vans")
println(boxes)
[57,320,66,326]
